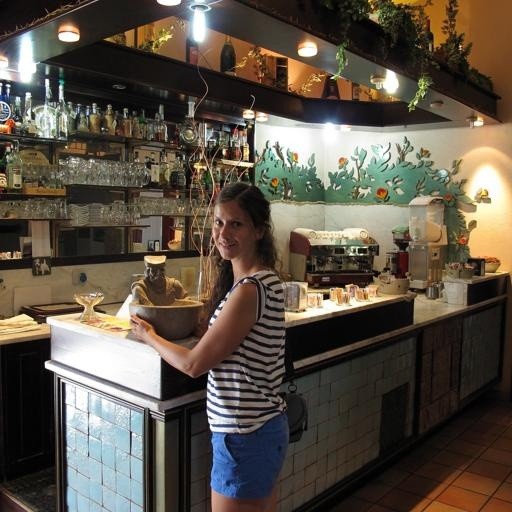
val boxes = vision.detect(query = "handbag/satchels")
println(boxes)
[282,333,305,443]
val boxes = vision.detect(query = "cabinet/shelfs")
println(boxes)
[1,69,255,271]
[0,299,125,474]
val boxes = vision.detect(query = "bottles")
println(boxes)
[220,34,237,74]
[0,141,58,190]
[160,93,253,204]
[185,24,201,66]
[349,82,362,101]
[0,79,168,143]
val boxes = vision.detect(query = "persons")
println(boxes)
[128,182,290,512]
[130,255,193,307]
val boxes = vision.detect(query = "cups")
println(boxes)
[308,284,378,307]
[105,197,208,224]
[0,198,69,221]
[60,154,151,186]
[427,286,439,299]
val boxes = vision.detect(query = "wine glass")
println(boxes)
[74,293,105,323]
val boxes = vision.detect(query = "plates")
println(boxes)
[70,204,104,226]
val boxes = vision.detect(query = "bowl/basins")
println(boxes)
[484,263,500,272]
[128,299,207,340]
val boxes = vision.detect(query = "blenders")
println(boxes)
[383,226,412,281]
